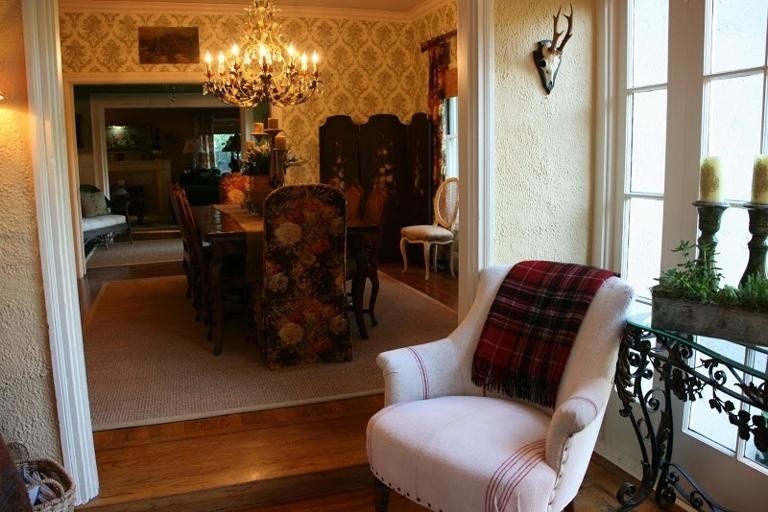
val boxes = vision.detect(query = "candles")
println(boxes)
[700,154,725,201]
[276,137,286,149]
[252,122,264,132]
[268,117,278,129]
[751,156,768,204]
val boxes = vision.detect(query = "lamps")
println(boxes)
[202,0,320,109]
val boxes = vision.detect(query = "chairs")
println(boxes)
[80,186,133,251]
[326,177,385,340]
[174,184,262,355]
[260,182,355,368]
[365,261,634,511]
[400,177,459,280]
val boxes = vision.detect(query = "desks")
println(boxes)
[190,201,379,341]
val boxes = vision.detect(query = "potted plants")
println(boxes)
[652,241,768,346]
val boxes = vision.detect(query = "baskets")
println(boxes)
[3,441,77,512]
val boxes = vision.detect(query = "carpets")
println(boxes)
[84,271,458,430]
[129,213,181,233]
[86,238,184,269]
